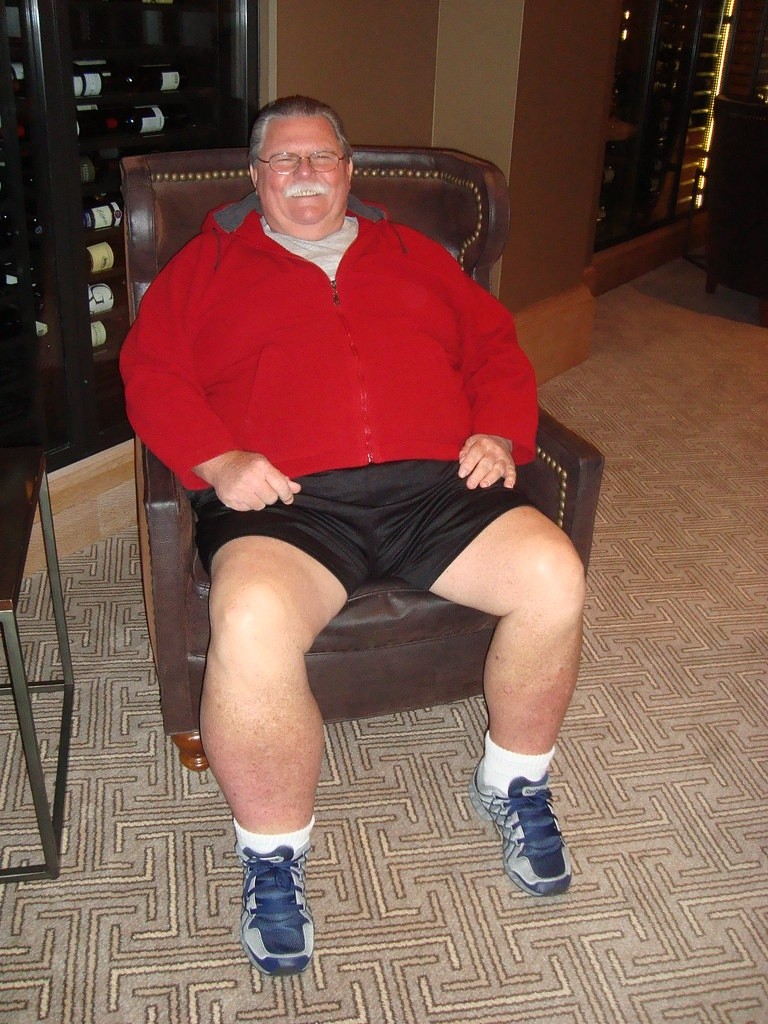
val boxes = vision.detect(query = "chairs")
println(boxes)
[0,445,76,886]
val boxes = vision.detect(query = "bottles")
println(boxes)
[0,62,186,354]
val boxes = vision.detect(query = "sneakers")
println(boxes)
[469,756,572,897]
[234,841,314,976]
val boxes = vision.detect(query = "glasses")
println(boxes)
[256,151,346,175]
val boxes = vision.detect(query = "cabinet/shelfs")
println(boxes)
[593,0,768,252]
[0,0,259,437]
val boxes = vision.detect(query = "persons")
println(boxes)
[119,95,587,976]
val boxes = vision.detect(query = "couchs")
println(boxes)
[702,92,768,298]
[119,147,604,771]
